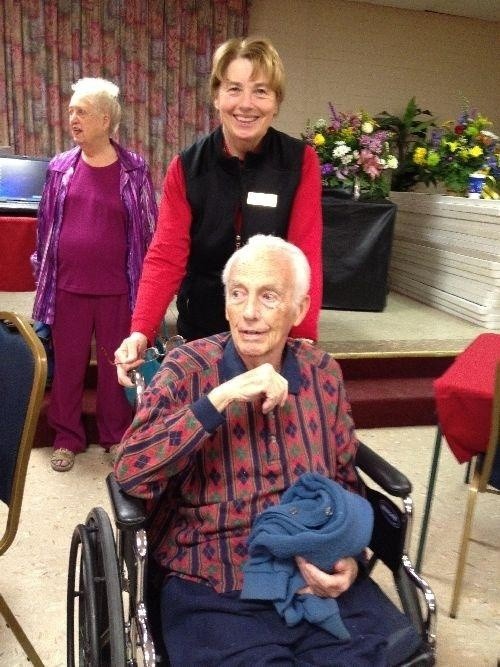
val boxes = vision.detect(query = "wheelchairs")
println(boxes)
[67,351,439,666]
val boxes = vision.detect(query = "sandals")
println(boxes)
[104,444,117,465]
[51,448,75,471]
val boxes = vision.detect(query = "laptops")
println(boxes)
[0,154,51,211]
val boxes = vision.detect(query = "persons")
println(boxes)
[30,78,159,472]
[115,39,324,386]
[116,234,432,667]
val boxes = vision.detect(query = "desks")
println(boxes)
[415,333,500,574]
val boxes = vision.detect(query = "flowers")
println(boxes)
[300,89,498,201]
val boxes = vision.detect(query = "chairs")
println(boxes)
[450,362,500,619]
[1,311,48,667]
